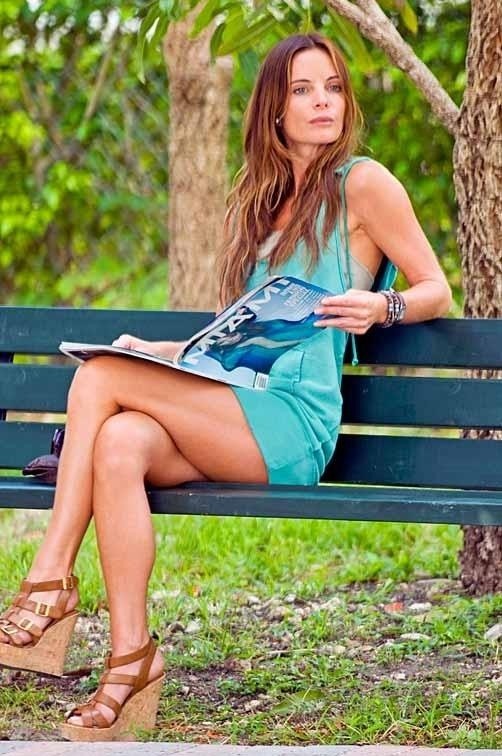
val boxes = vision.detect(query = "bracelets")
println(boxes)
[379,286,406,330]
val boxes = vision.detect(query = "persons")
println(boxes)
[0,29,454,743]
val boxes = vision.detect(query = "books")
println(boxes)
[57,274,341,389]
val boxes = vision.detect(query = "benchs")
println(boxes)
[0,306,501,529]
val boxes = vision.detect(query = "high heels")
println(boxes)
[60,634,166,742]
[0,573,81,679]
